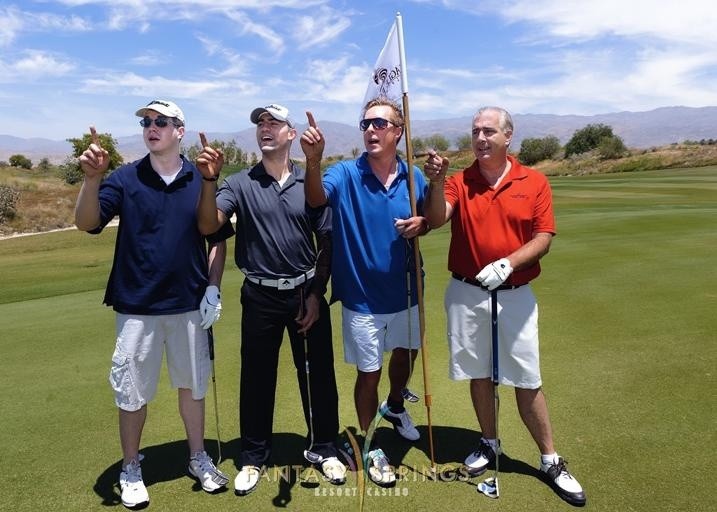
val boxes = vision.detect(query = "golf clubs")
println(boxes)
[477,287,499,499]
[204,309,223,470]
[299,282,322,465]
[399,238,421,402]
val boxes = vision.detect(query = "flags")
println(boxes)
[359,16,408,120]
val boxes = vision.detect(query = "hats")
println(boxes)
[136,100,186,126]
[251,104,296,129]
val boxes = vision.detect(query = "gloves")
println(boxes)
[478,258,513,291]
[200,286,222,329]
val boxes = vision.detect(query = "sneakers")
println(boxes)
[233,466,260,495]
[381,400,421,441]
[120,461,150,508]
[319,456,346,485]
[362,447,397,487]
[540,453,587,506]
[188,449,227,492]
[465,437,503,476]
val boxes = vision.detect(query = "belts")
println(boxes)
[449,271,519,292]
[247,268,315,289]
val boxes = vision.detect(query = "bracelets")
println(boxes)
[202,174,219,181]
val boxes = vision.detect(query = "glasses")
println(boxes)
[360,119,396,130]
[139,118,177,127]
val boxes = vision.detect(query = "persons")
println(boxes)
[75,101,229,508]
[424,107,586,507]
[300,98,432,487]
[198,104,346,496]
